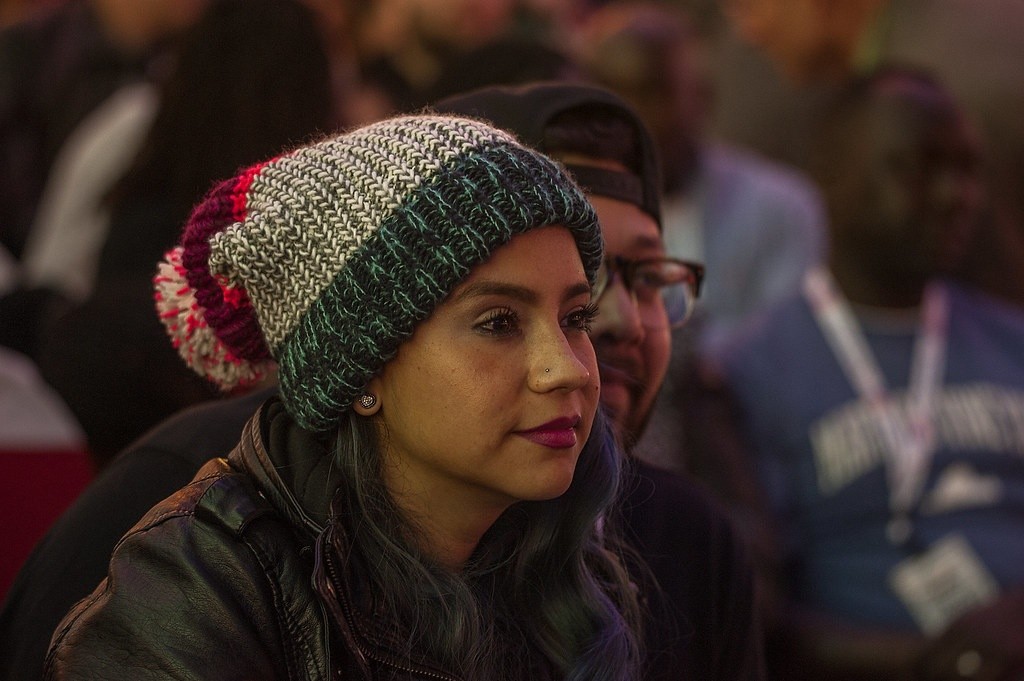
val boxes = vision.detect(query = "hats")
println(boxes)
[152,112,606,431]
[431,85,665,226]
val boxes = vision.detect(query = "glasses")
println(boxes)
[584,256,709,330]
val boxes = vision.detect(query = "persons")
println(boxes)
[565,15,832,481]
[0,82,763,681]
[0,0,570,609]
[44,112,649,681]
[686,63,1024,681]
